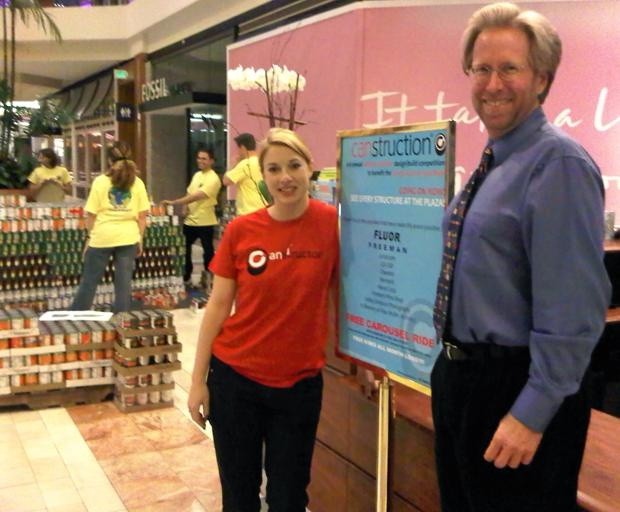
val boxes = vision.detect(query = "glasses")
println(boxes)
[470,62,528,80]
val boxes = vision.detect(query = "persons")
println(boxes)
[161,145,222,290]
[72,141,150,312]
[223,130,270,216]
[27,144,73,203]
[190,127,376,511]
[430,0,612,511]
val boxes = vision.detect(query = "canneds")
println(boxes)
[0,194,187,408]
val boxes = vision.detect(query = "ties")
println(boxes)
[433,146,492,343]
